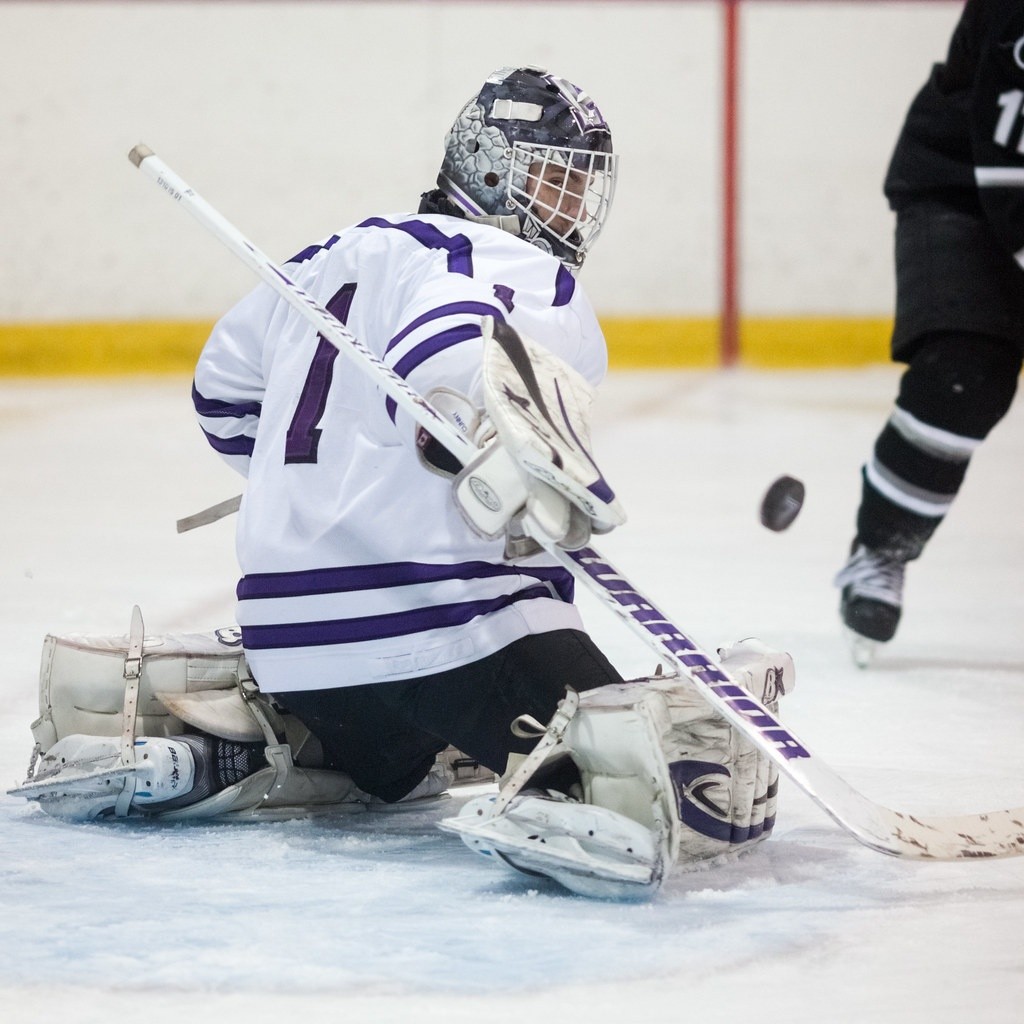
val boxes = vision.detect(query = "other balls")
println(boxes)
[757,474,805,532]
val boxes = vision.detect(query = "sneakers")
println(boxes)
[833,536,906,668]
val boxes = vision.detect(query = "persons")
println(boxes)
[6,67,793,902]
[833,1,1024,643]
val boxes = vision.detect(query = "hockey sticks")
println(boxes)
[127,141,1024,863]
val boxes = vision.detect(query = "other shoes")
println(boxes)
[34,723,257,822]
[460,796,664,899]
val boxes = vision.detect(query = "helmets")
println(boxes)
[436,59,612,268]
